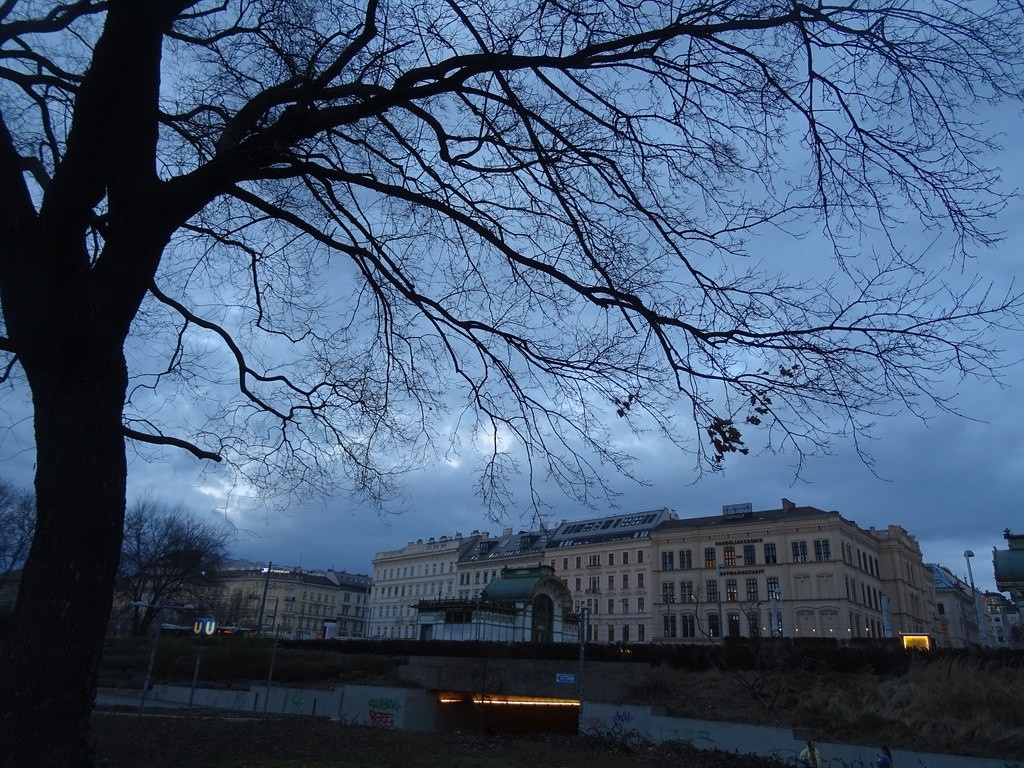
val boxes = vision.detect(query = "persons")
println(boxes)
[799,739,820,768]
[877,745,894,768]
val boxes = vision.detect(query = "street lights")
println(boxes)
[963,548,987,649]
[132,600,197,720]
[473,600,490,640]
[576,603,589,717]
[774,588,784,637]
[716,562,727,638]
